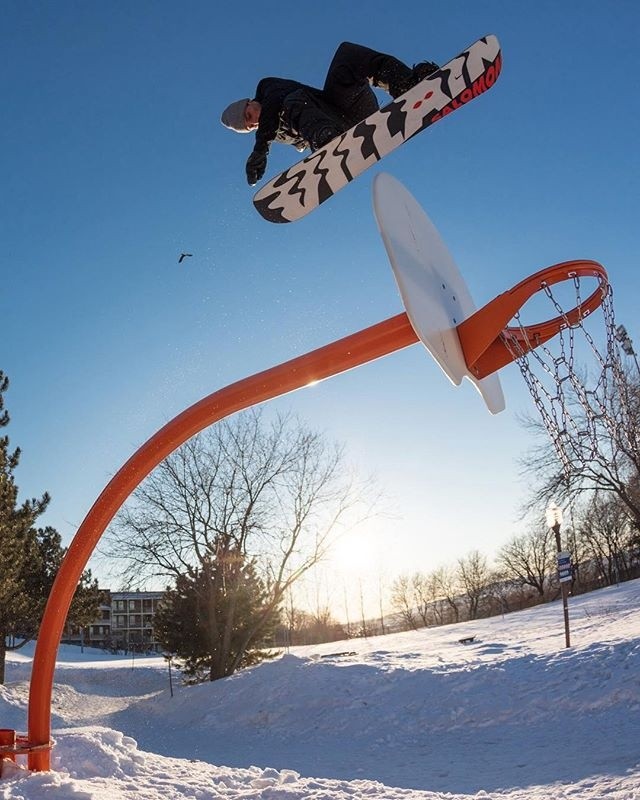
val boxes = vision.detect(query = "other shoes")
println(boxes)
[292,106,343,153]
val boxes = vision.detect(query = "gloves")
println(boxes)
[246,151,268,187]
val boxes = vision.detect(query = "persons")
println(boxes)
[221,43,440,188]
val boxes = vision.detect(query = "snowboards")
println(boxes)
[250,31,506,225]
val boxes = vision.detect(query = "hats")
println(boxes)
[220,98,250,133]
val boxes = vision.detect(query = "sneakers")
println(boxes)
[374,56,439,99]
